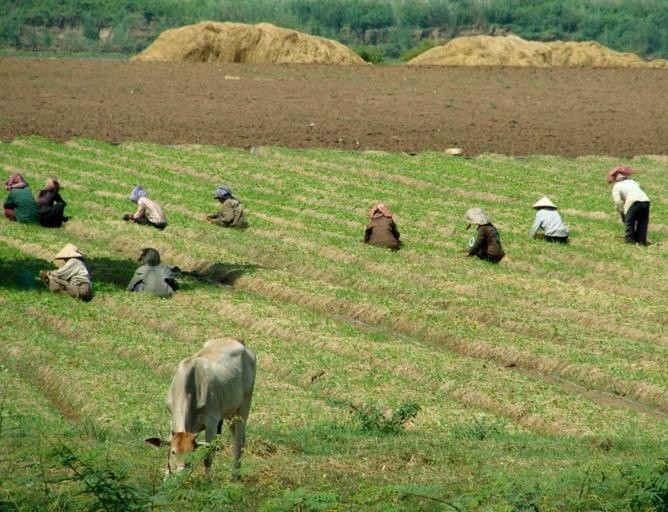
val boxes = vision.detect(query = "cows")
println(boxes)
[142,334,258,492]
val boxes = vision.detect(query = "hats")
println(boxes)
[213,189,231,200]
[533,197,556,208]
[464,208,490,224]
[55,243,83,258]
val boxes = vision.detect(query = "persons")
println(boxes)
[125,248,181,298]
[463,207,505,264]
[4,173,37,223]
[606,165,650,245]
[123,183,167,230]
[529,196,571,243]
[207,187,248,227]
[36,242,91,299]
[36,179,67,226]
[364,204,400,250]
